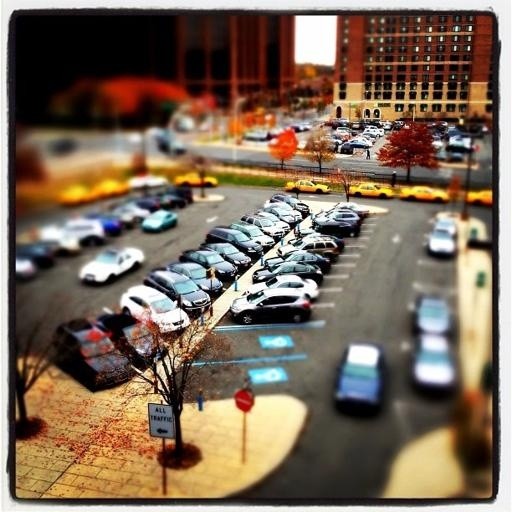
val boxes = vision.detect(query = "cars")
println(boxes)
[243,274,320,304]
[252,262,324,286]
[323,118,411,154]
[178,193,308,279]
[413,294,454,338]
[244,121,312,142]
[97,313,169,366]
[281,175,493,207]
[427,217,459,258]
[167,262,224,294]
[426,121,489,162]
[15,172,220,284]
[286,201,369,251]
[410,335,457,394]
[333,342,388,416]
[264,251,331,276]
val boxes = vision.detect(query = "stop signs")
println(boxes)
[235,391,253,411]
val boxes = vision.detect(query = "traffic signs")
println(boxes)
[147,403,176,439]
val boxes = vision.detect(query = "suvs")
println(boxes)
[50,318,132,392]
[276,237,340,263]
[143,266,212,315]
[230,287,312,325]
[120,284,191,333]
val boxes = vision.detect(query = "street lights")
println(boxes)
[232,97,250,159]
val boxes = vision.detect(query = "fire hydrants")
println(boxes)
[192,388,213,412]
